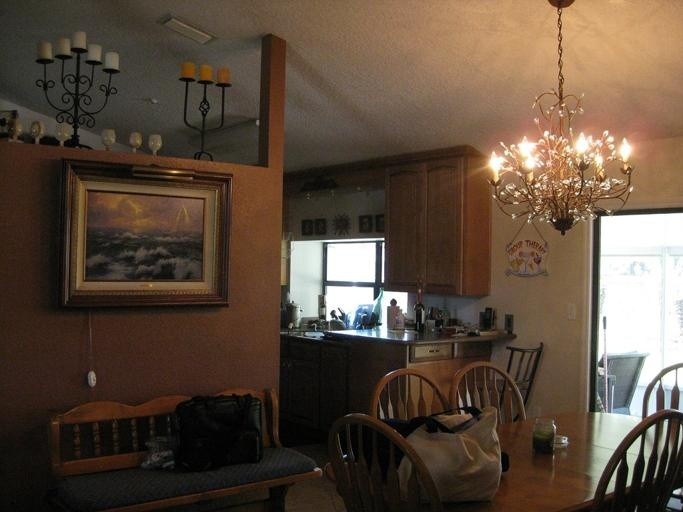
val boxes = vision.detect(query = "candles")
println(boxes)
[71,31,86,48]
[36,42,52,59]
[200,64,212,80]
[55,38,72,55]
[103,51,120,69]
[181,62,195,78]
[218,66,230,82]
[87,44,101,60]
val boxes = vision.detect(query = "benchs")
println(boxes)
[48,387,323,512]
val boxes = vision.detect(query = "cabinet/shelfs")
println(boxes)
[319,340,349,428]
[280,332,322,426]
[349,340,492,420]
[385,157,493,296]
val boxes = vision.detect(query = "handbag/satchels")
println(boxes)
[399,406,502,503]
[174,394,261,470]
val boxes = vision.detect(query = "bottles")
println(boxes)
[414,288,426,332]
[319,295,326,322]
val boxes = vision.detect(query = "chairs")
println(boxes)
[597,351,650,416]
[471,342,544,423]
[448,362,527,424]
[370,369,451,421]
[327,414,443,512]
[642,362,683,512]
[590,408,683,512]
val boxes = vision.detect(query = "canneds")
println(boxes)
[532,417,557,453]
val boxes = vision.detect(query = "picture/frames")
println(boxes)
[56,155,234,307]
[359,214,373,233]
[375,214,384,232]
[314,218,326,235]
[301,220,313,236]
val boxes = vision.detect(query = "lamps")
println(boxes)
[486,0,636,233]
[156,10,219,47]
